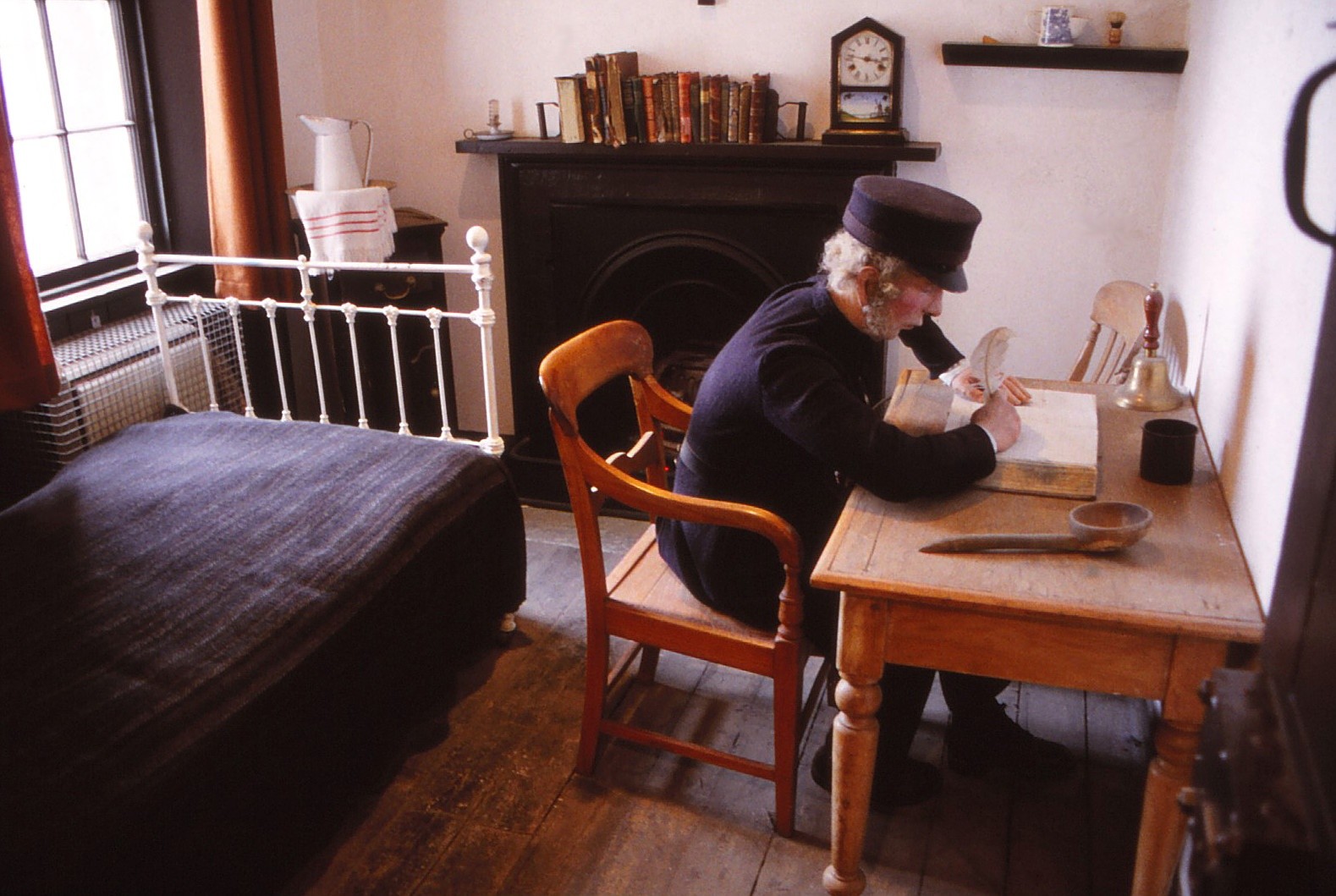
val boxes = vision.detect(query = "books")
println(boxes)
[554,51,771,149]
[944,387,1098,501]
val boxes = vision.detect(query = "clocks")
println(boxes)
[821,16,910,145]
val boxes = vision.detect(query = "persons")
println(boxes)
[655,176,1079,809]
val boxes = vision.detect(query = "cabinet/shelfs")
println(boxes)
[292,207,460,438]
[456,136,942,521]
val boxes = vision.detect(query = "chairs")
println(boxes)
[1067,280,1149,385]
[538,320,840,836]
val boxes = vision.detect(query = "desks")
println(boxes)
[810,370,1266,896]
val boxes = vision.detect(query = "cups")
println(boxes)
[1139,419,1198,484]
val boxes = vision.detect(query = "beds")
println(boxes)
[0,220,514,896]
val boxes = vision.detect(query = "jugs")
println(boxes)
[299,113,373,191]
[1027,6,1088,47]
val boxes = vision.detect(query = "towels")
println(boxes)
[291,185,399,277]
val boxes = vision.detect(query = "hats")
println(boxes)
[841,176,982,293]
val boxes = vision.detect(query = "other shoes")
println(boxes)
[943,714,1076,780]
[809,734,942,809]
